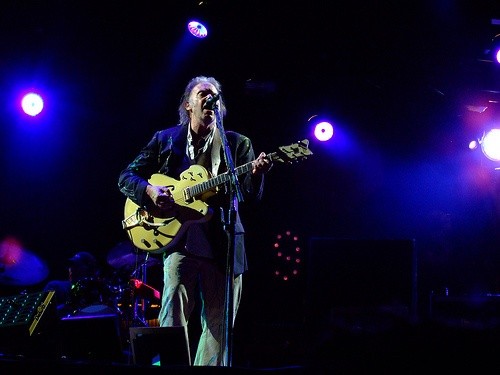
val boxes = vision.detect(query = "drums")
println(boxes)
[70,275,109,313]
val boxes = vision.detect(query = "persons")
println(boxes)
[44,252,97,306]
[119,76,272,367]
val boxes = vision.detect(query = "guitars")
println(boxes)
[123,138,313,252]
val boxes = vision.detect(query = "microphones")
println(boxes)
[206,91,221,106]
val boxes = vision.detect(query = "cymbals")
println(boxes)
[105,240,162,274]
[0,243,49,287]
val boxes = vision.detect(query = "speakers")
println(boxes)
[305,237,418,342]
